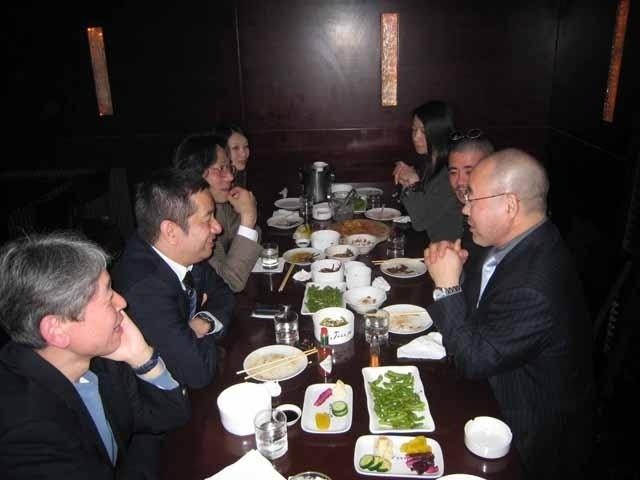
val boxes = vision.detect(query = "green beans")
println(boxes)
[368,371,425,430]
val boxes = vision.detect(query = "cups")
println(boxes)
[260,272,279,299]
[260,244,279,269]
[273,311,300,345]
[298,160,383,220]
[254,409,289,460]
[365,310,390,345]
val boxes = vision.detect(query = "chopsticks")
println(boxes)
[234,348,318,381]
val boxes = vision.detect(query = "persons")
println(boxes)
[107,158,238,392]
[1,226,195,480]
[391,97,467,246]
[214,119,255,195]
[421,148,605,480]
[445,126,498,204]
[173,129,267,294]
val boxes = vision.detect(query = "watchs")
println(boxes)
[432,282,463,302]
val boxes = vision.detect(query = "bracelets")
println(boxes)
[132,346,160,378]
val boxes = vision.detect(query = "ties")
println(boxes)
[477,257,496,300]
[183,272,199,317]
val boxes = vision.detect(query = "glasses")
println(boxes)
[462,193,502,208]
[206,165,236,178]
[449,127,483,141]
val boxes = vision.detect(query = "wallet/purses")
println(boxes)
[254,294,283,314]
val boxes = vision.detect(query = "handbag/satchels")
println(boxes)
[381,180,406,206]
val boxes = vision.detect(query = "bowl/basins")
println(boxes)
[217,383,273,437]
[330,342,355,363]
[273,404,301,427]
[311,305,355,346]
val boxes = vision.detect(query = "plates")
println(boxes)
[366,207,403,222]
[376,304,433,336]
[283,229,428,315]
[354,364,487,480]
[299,382,353,434]
[267,196,305,228]
[242,343,309,381]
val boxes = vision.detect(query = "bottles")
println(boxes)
[369,334,380,365]
[316,326,333,378]
